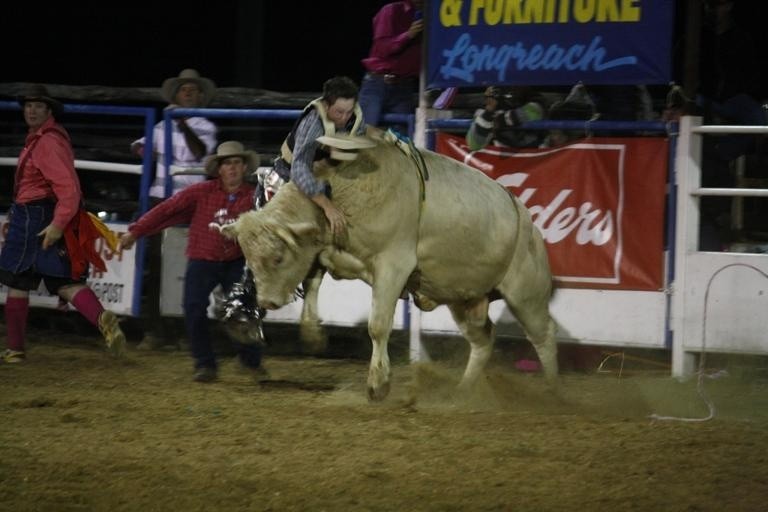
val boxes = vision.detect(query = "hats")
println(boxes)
[316,133,377,162]
[483,85,513,100]
[16,84,65,112]
[204,141,261,176]
[159,70,218,106]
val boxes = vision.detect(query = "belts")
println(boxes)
[373,70,402,85]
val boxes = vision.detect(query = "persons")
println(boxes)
[0,82,127,365]
[464,84,548,153]
[355,1,424,128]
[118,138,272,384]
[216,75,367,350]
[127,68,221,352]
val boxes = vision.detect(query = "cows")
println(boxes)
[207,123,561,405]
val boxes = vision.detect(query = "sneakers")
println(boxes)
[244,362,270,383]
[98,313,126,355]
[175,333,193,351]
[135,335,160,351]
[193,364,216,381]
[0,347,26,364]
